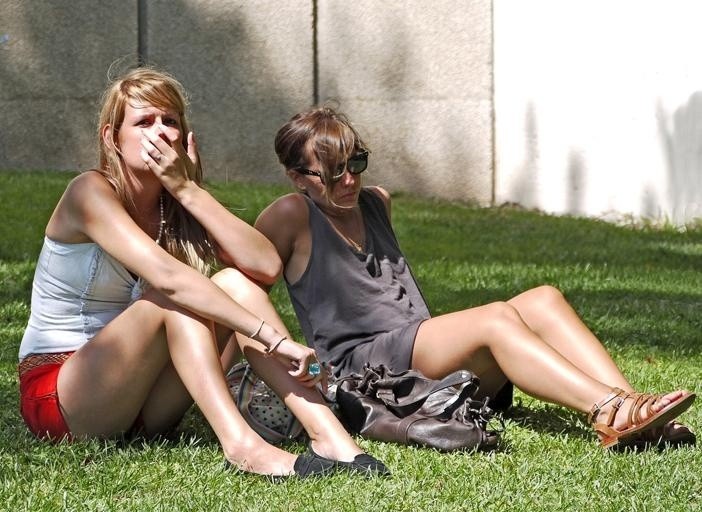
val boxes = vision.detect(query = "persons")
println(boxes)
[18,68,391,484]
[253,106,696,451]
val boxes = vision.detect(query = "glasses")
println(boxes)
[294,152,368,183]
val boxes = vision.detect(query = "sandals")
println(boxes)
[587,387,696,449]
[225,439,393,483]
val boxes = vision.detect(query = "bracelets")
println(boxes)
[264,335,287,356]
[249,320,264,339]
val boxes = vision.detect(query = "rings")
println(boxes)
[309,363,321,375]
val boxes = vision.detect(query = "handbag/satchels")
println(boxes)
[226,363,497,452]
